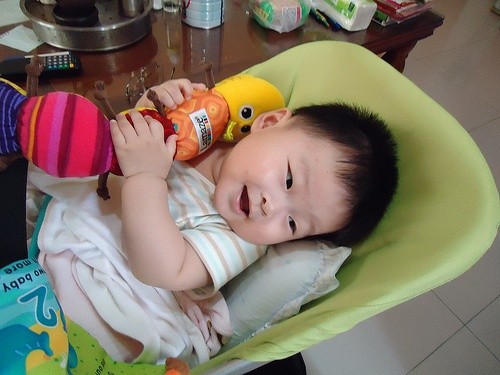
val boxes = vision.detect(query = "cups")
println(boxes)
[162,1,179,12]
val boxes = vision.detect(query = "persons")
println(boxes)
[26,78,399,371]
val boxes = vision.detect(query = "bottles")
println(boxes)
[118,0,145,17]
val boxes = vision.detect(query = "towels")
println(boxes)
[29,166,235,365]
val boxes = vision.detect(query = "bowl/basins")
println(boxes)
[19,0,153,52]
